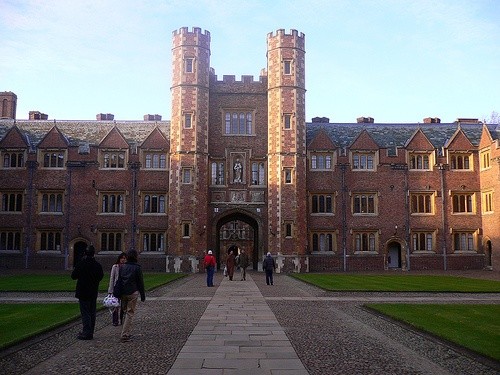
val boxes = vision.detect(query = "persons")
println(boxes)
[221,253,229,277]
[71,245,104,340]
[263,252,275,285]
[234,158,242,182]
[119,249,145,342]
[108,252,127,326]
[205,250,216,287]
[226,251,236,281]
[237,249,248,281]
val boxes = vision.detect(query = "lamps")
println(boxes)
[200,224,207,235]
[269,224,276,236]
[395,224,398,236]
[77,223,81,233]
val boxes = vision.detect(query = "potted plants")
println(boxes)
[388,256,392,266]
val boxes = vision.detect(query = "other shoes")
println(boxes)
[271,282,273,285]
[121,335,132,343]
[267,282,269,285]
[240,279,243,281]
[79,329,83,334]
[78,334,93,340]
[112,322,118,326]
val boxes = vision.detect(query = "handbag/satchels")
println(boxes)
[103,294,119,315]
[224,267,227,277]
